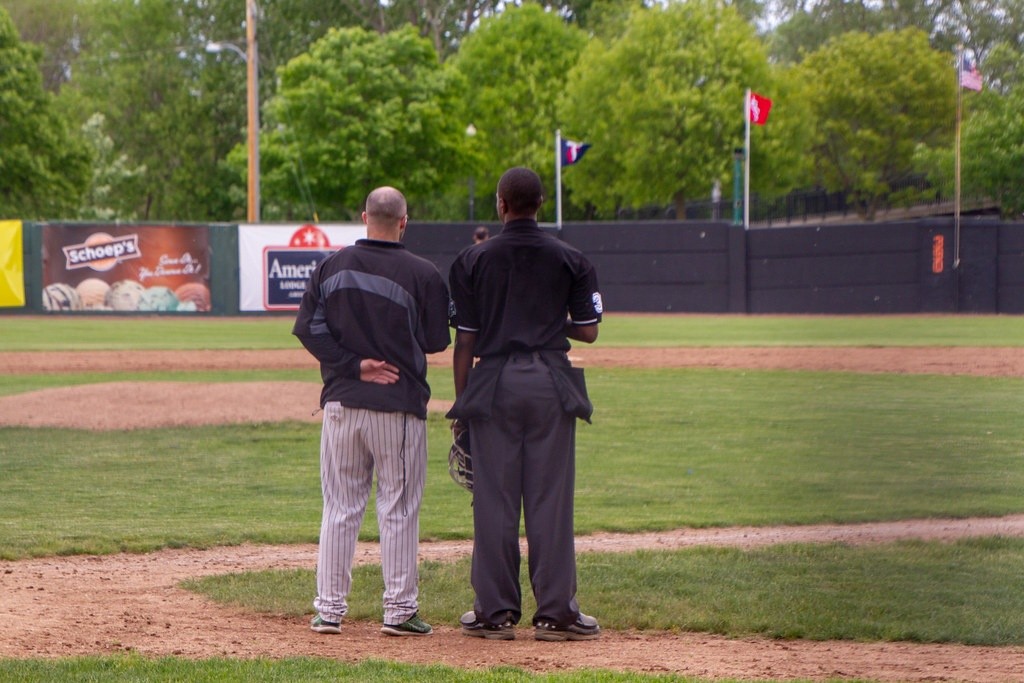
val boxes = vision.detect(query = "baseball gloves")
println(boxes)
[447,417,473,494]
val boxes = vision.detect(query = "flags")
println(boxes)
[745,91,772,126]
[560,137,593,167]
[959,52,982,91]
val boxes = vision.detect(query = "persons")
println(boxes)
[290,186,451,636]
[443,168,601,643]
[471,227,488,243]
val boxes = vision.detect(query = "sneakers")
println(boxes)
[536,612,599,641]
[380,609,433,635]
[309,613,342,633]
[460,611,515,640]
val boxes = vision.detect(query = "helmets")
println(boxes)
[447,430,474,495]
